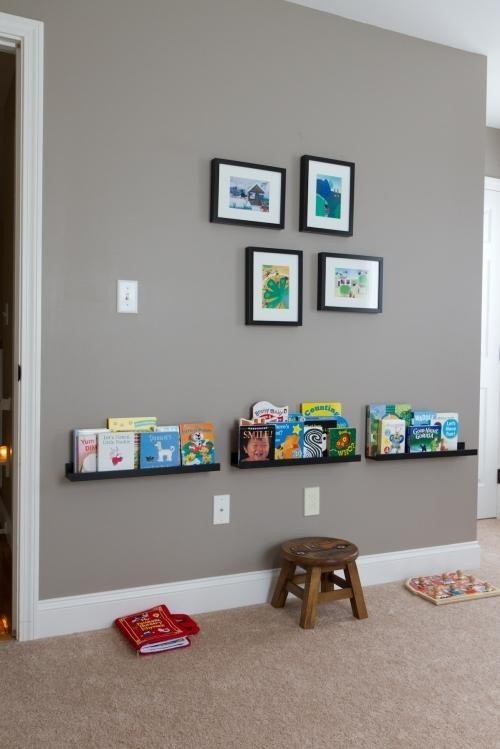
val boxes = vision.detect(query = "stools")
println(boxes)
[274,533,368,632]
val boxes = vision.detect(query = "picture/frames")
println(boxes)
[239,245,303,328]
[299,151,356,239]
[210,155,288,231]
[316,251,384,313]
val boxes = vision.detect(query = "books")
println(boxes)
[366,403,460,457]
[238,402,356,462]
[115,604,193,656]
[73,416,216,474]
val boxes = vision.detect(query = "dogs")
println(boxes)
[154,441,175,461]
